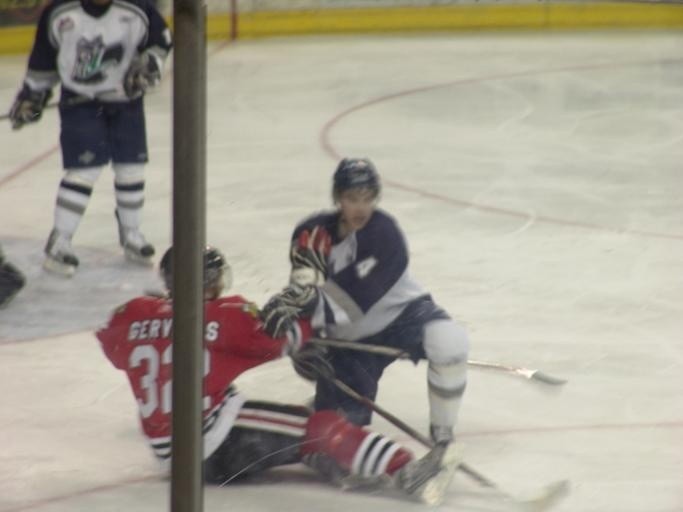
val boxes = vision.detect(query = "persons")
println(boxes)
[259,158,466,447]
[96,243,465,504]
[10,0,175,279]
[0,254,27,307]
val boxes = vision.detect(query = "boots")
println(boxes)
[43,228,79,265]
[118,223,155,256]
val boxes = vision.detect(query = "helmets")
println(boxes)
[159,243,233,299]
[332,157,382,206]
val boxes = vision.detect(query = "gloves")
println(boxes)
[260,282,337,382]
[123,54,161,100]
[8,82,54,131]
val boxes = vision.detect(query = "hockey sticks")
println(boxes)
[318,363,572,508]
[309,338,566,385]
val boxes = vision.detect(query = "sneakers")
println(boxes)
[306,450,352,479]
[398,441,449,498]
[430,416,456,446]
